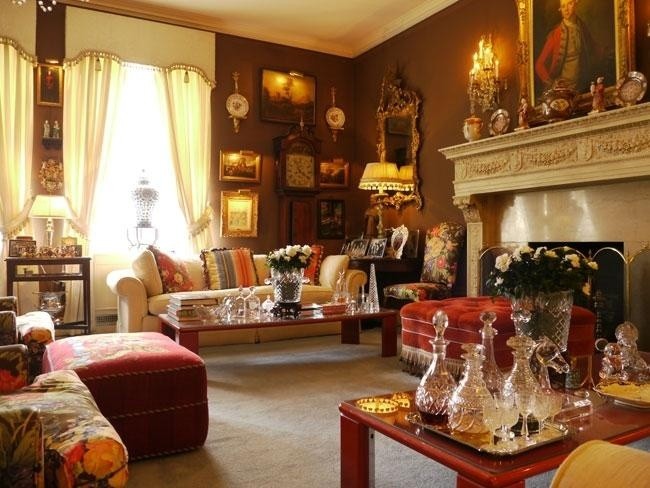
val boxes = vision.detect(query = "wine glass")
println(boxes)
[481,390,563,453]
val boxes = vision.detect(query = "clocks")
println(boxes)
[271,113,324,251]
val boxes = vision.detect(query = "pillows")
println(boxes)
[132,250,164,297]
[147,245,196,293]
[199,245,261,290]
[303,244,325,287]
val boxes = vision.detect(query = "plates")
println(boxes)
[613,69,648,106]
[593,387,649,409]
[226,93,250,116]
[487,109,510,135]
[325,107,346,127]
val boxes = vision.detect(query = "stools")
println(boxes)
[398,292,598,384]
[41,331,211,464]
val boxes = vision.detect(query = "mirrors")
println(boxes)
[368,57,425,213]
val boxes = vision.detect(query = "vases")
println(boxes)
[264,266,311,319]
[511,283,574,353]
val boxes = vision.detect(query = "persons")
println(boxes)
[517,97,528,127]
[45,71,57,100]
[535,0,601,98]
[590,76,606,110]
[352,240,384,256]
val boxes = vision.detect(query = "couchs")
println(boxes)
[104,243,369,348]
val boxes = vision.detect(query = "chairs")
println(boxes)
[1,342,130,486]
[1,293,56,388]
[381,220,466,309]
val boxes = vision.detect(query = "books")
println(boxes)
[166,293,218,322]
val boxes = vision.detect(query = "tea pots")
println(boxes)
[462,116,484,142]
[539,79,581,124]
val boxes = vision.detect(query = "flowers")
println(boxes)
[264,242,315,272]
[484,239,602,299]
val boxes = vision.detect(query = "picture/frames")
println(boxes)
[317,197,346,241]
[258,64,318,129]
[36,64,64,108]
[514,0,635,127]
[7,235,83,257]
[218,147,262,184]
[219,187,260,240]
[386,117,410,138]
[319,154,351,193]
[339,225,420,260]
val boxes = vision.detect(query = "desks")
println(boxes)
[158,305,398,357]
[4,256,94,335]
[348,256,423,306]
[338,349,650,486]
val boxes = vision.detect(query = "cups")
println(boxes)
[71,245,82,258]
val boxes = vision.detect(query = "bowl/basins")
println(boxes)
[192,304,228,323]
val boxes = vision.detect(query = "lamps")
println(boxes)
[27,193,73,250]
[466,30,509,119]
[399,165,415,191]
[359,160,401,238]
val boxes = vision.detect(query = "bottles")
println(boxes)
[416,309,459,432]
[223,285,274,318]
[503,332,539,391]
[43,119,50,138]
[447,342,496,434]
[478,310,506,396]
[53,121,60,138]
[333,263,380,315]
